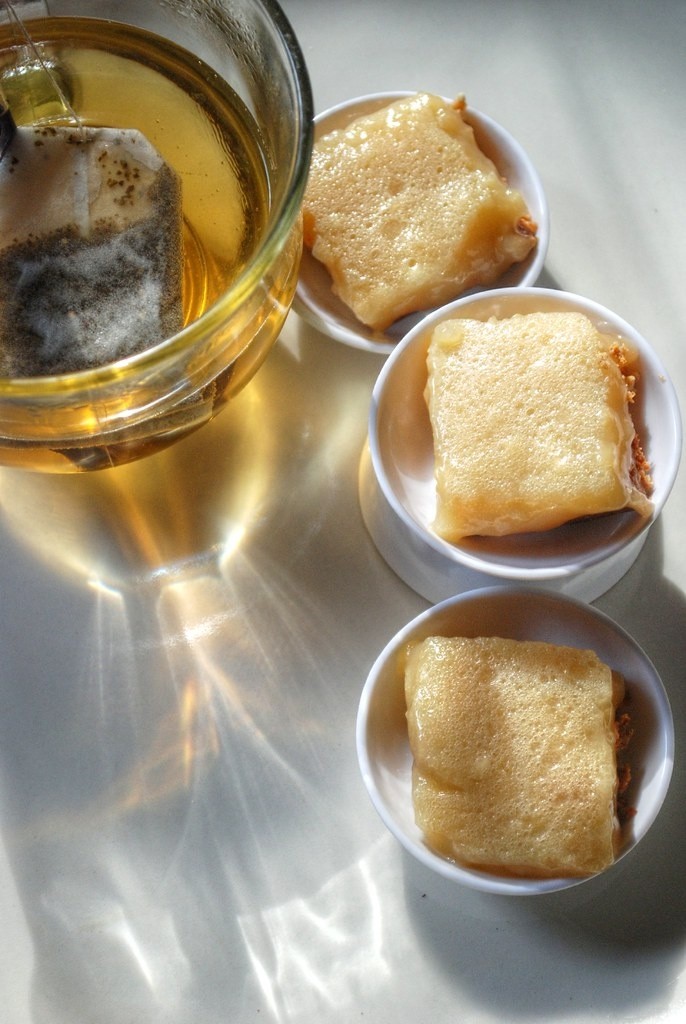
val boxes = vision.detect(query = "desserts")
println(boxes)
[419,309,654,541]
[403,634,635,879]
[298,89,540,334]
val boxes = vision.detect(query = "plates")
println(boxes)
[355,583,678,895]
[278,92,546,353]
[369,289,683,580]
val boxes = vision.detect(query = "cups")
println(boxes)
[0,1,314,473]
